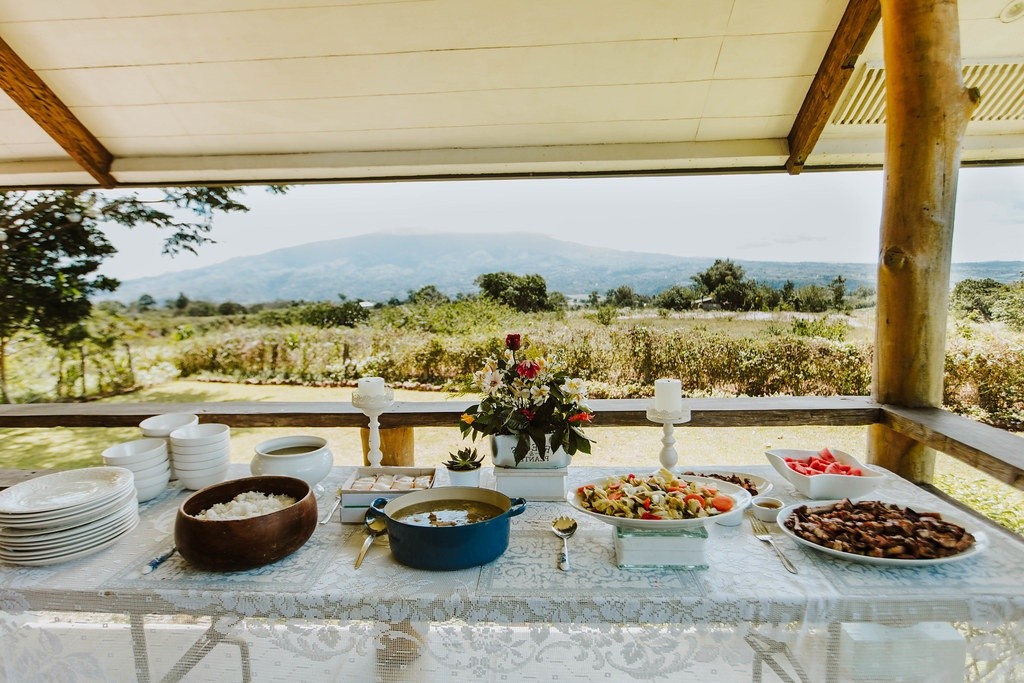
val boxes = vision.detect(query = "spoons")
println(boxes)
[551,516,577,571]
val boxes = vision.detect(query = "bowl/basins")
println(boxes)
[102,414,230,503]
[715,509,743,526]
[764,449,885,500]
[174,475,318,572]
[752,497,785,522]
[250,435,333,486]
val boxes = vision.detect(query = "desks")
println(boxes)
[0,465,1023,682]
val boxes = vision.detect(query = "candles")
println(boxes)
[655,378,681,413]
[359,377,385,396]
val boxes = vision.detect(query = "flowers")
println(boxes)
[459,334,597,467]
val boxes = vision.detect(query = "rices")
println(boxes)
[188,490,297,520]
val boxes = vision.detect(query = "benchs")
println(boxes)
[0,396,1024,492]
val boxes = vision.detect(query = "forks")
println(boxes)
[750,517,798,574]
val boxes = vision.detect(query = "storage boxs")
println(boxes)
[340,467,436,526]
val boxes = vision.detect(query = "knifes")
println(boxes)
[319,495,341,525]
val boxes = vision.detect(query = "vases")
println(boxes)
[491,435,572,468]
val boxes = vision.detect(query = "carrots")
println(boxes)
[577,473,733,511]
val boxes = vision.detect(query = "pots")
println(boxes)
[370,485,527,571]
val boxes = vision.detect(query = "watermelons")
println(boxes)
[783,447,862,475]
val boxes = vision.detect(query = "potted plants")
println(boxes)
[442,447,486,487]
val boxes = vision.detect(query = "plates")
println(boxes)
[681,471,774,499]
[566,474,752,529]
[0,467,139,566]
[777,501,987,565]
